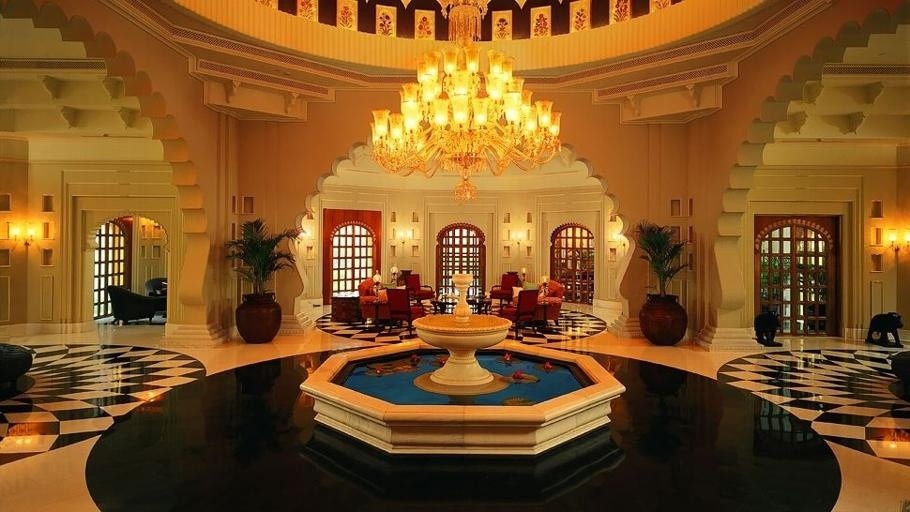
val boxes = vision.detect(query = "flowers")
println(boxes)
[410,354,419,364]
[374,364,383,374]
[543,361,553,369]
[502,353,513,361]
[512,370,524,379]
[438,356,448,365]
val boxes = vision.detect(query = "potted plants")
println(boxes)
[798,247,827,330]
[760,255,791,313]
[631,215,689,346]
[223,218,302,343]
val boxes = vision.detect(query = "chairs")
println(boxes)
[406,274,435,303]
[387,289,424,333]
[490,272,522,308]
[358,278,387,322]
[501,290,538,333]
[106,285,157,323]
[513,280,566,325]
[145,278,167,313]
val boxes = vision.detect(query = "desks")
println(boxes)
[431,295,492,316]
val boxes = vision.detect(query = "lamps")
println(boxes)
[888,226,910,253]
[366,0,558,201]
[8,224,38,245]
